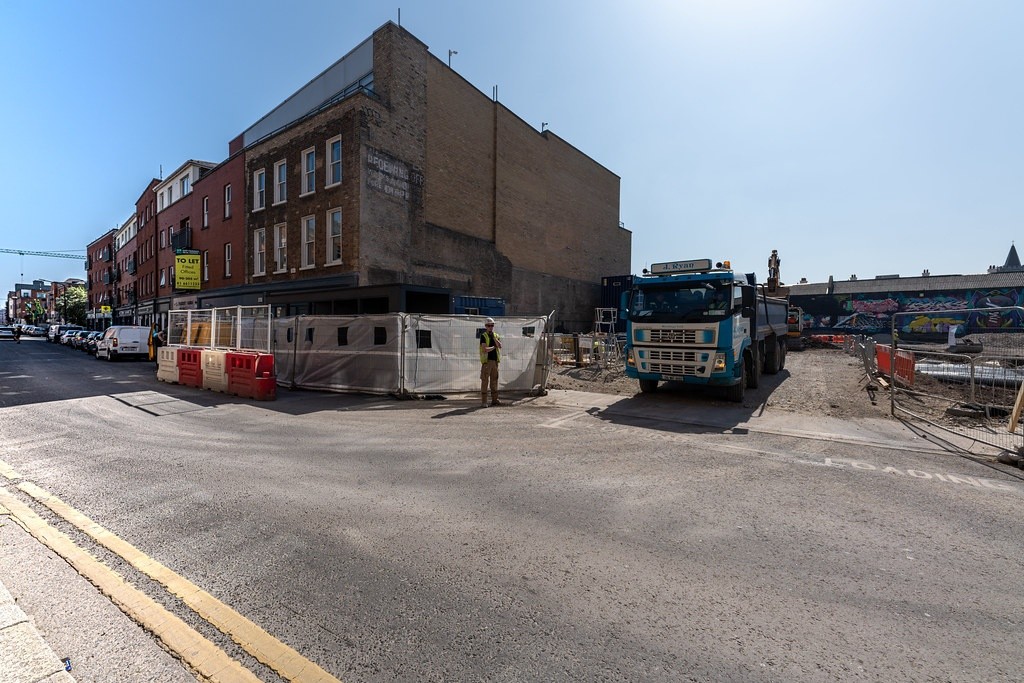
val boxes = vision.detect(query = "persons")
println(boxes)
[479,317,505,407]
[14,327,21,344]
[788,314,796,324]
[157,319,186,346]
[894,329,899,348]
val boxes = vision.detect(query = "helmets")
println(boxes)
[485,318,494,324]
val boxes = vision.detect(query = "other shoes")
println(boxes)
[482,403,488,407]
[491,400,505,406]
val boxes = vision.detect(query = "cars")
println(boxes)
[0,323,151,361]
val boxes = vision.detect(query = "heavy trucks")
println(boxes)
[622,259,788,403]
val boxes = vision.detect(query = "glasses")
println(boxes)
[485,325,494,327]
